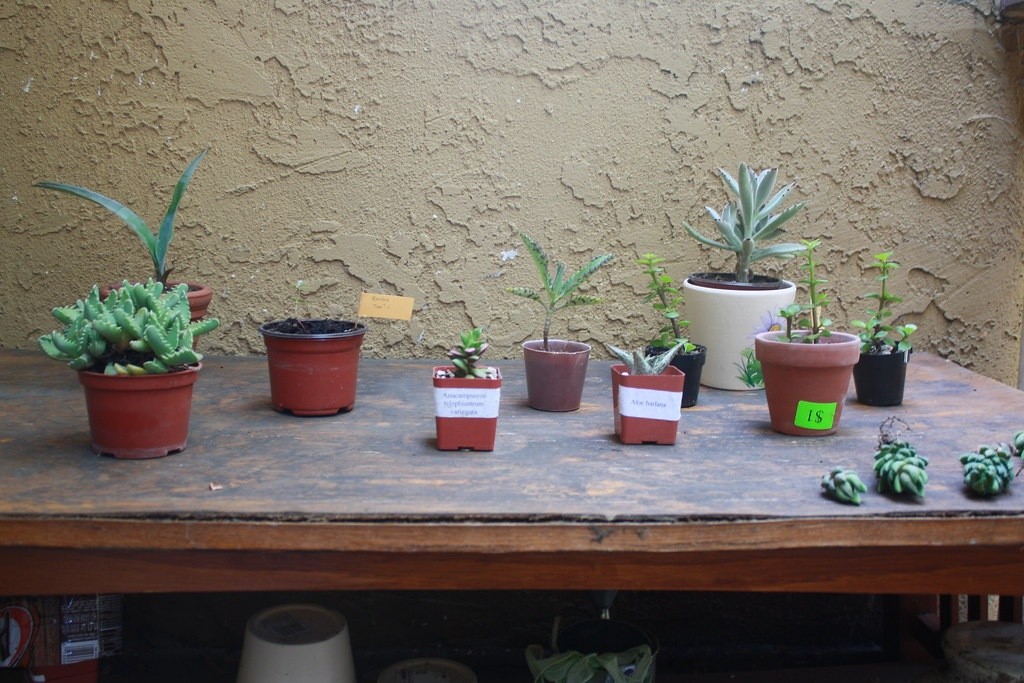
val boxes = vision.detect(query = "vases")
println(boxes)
[258,315,368,415]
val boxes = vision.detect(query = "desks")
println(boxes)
[1,337,1024,683]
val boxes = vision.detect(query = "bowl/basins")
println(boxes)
[235,603,356,683]
[375,657,478,683]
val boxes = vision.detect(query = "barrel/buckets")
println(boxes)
[547,617,660,683]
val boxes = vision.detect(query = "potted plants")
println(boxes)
[503,233,615,412]
[605,339,690,446]
[36,276,222,459]
[30,148,215,354]
[635,253,707,408]
[755,237,860,436]
[849,251,917,407]
[683,161,812,392]
[525,613,660,683]
[430,328,502,453]
